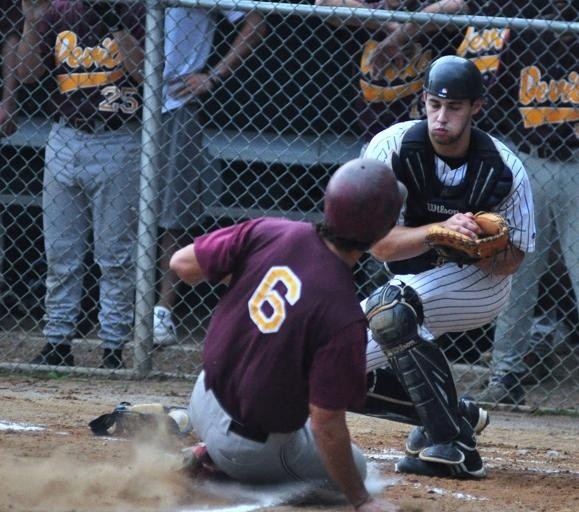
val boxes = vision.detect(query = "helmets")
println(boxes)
[423,54,482,99]
[324,158,403,245]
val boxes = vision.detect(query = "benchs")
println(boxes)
[0,114,368,225]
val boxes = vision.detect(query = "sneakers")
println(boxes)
[473,377,525,405]
[153,306,177,346]
[29,342,74,369]
[405,398,489,454]
[98,349,127,369]
[395,440,487,479]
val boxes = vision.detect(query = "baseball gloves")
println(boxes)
[426,211,524,275]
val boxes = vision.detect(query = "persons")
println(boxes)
[0,1,21,139]
[14,1,148,367]
[463,0,579,405]
[351,49,537,476]
[150,1,270,351]
[86,155,408,511]
[315,1,468,137]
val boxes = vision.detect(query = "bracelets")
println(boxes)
[205,67,217,89]
